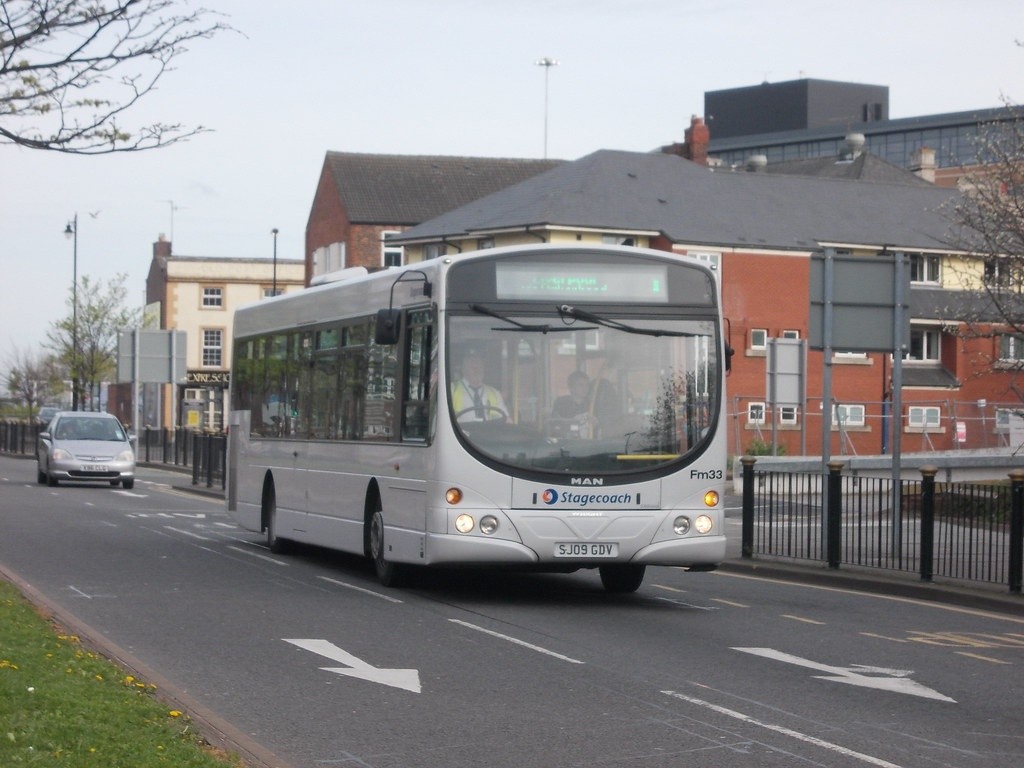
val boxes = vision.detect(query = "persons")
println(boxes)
[551,372,590,419]
[63,423,78,440]
[450,350,514,425]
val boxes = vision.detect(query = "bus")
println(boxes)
[225,242,736,596]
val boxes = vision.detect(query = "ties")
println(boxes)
[473,386,483,420]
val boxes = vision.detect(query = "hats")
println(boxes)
[459,342,486,361]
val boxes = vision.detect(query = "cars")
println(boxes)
[37,411,138,489]
[35,407,61,425]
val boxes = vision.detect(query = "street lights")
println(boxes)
[536,58,559,158]
[63,220,77,413]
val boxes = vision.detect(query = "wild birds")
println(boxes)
[88,210,100,219]
